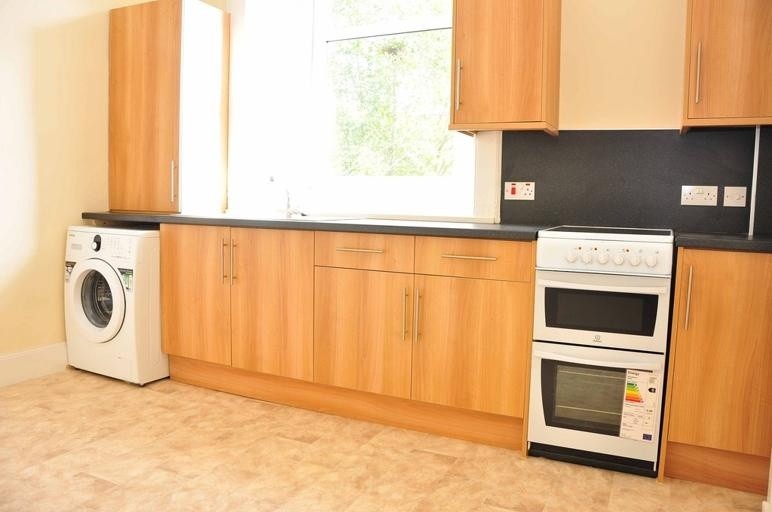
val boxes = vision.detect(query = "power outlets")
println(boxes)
[723,186,747,207]
[682,186,718,208]
[504,182,535,200]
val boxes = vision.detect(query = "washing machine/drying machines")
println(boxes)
[61,226,169,387]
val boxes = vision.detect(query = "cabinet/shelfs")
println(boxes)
[110,1,231,214]
[313,229,533,456]
[160,223,313,410]
[681,1,771,134]
[659,231,772,492]
[448,0,560,138]
